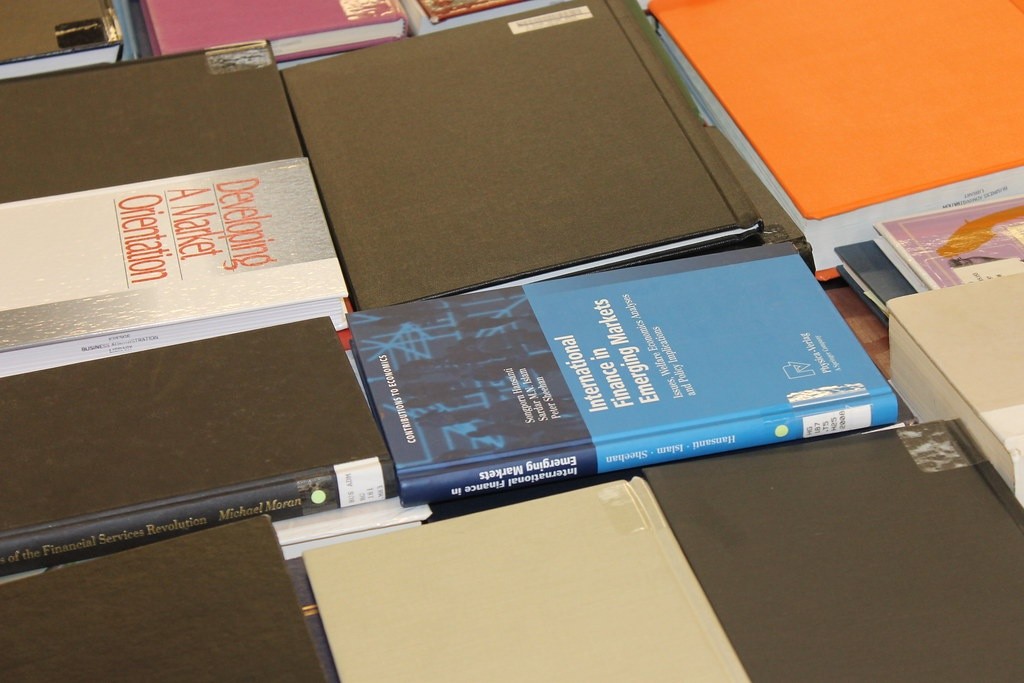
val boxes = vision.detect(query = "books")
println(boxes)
[0,0,1024,683]
